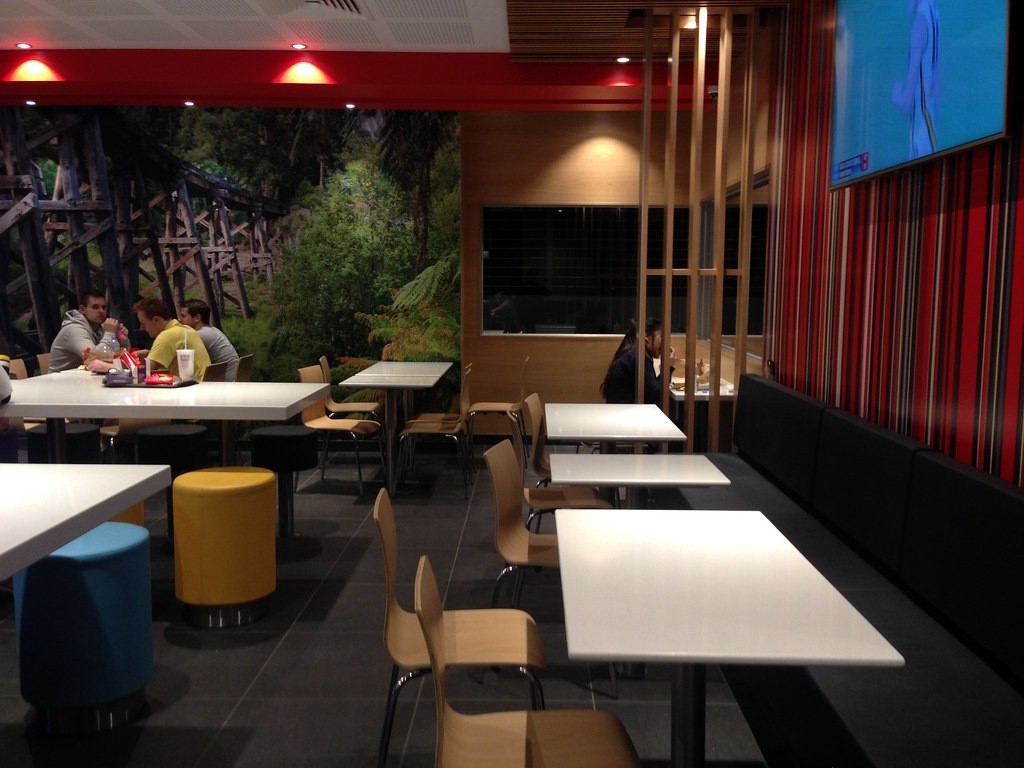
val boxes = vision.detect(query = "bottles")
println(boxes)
[115,325,131,350]
[109,336,120,350]
[695,364,698,394]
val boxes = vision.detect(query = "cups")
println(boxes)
[679,359,685,378]
[100,346,113,364]
[176,349,196,378]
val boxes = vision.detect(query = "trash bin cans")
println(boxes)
[15,520,154,745]
[165,465,285,633]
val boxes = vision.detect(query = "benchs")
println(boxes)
[677,373,1024,768]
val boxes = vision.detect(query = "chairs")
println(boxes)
[5,352,255,464]
[298,355,384,499]
[373,362,646,768]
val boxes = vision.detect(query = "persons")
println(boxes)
[46,289,128,464]
[0,330,13,433]
[601,317,676,454]
[179,299,240,382]
[88,296,207,464]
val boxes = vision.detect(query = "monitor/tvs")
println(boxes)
[824,0,1015,194]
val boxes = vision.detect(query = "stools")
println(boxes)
[13,422,318,738]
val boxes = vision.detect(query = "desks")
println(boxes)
[553,507,906,768]
[0,372,332,563]
[666,374,739,451]
[543,399,689,505]
[339,357,455,504]
[0,464,174,584]
[544,448,735,511]
